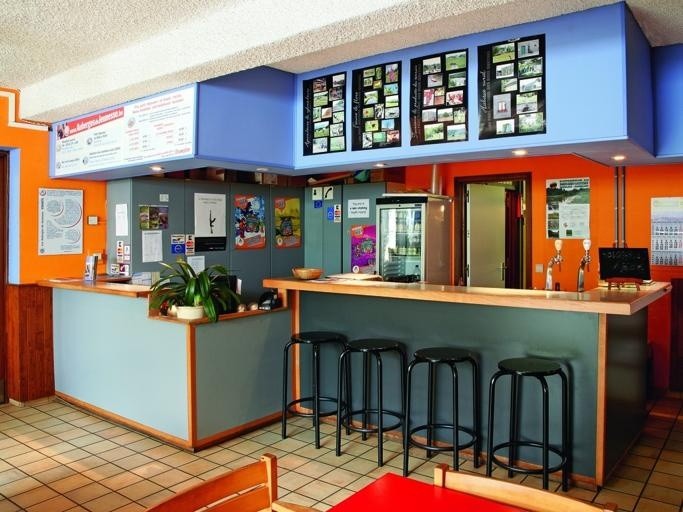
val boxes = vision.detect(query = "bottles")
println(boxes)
[380,210,420,234]
[381,236,420,257]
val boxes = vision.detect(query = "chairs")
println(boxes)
[432,463,609,511]
[146,451,323,512]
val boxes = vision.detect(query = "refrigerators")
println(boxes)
[374,192,452,284]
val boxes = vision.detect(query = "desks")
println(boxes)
[325,472,530,511]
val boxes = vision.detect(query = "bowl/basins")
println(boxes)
[290,266,323,281]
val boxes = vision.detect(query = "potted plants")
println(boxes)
[150,258,240,324]
